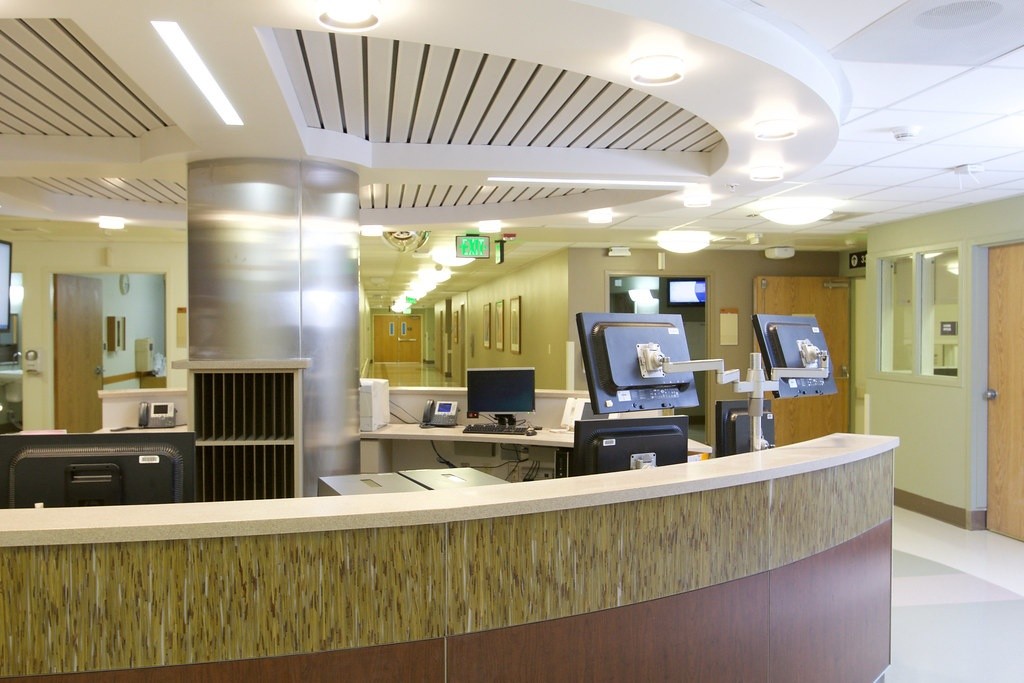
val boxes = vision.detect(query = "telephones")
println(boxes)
[138,402,175,428]
[420,400,458,428]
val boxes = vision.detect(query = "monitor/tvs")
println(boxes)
[752,314,838,400]
[668,278,706,307]
[0,431,197,510]
[715,400,775,459]
[574,415,688,477]
[0,239,12,332]
[466,367,536,426]
[575,312,700,415]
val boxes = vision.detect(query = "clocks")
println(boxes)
[119,273,130,295]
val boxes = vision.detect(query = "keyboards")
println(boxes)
[463,424,527,435]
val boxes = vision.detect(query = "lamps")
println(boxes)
[657,230,709,254]
[764,246,795,259]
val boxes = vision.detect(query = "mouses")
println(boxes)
[526,428,537,436]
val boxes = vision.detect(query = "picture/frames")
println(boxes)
[494,299,504,351]
[510,296,521,354]
[452,311,458,343]
[482,302,491,349]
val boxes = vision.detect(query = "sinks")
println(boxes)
[0,370,23,385]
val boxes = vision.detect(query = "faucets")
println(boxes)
[12,352,22,368]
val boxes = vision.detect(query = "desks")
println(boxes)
[358,424,713,474]
